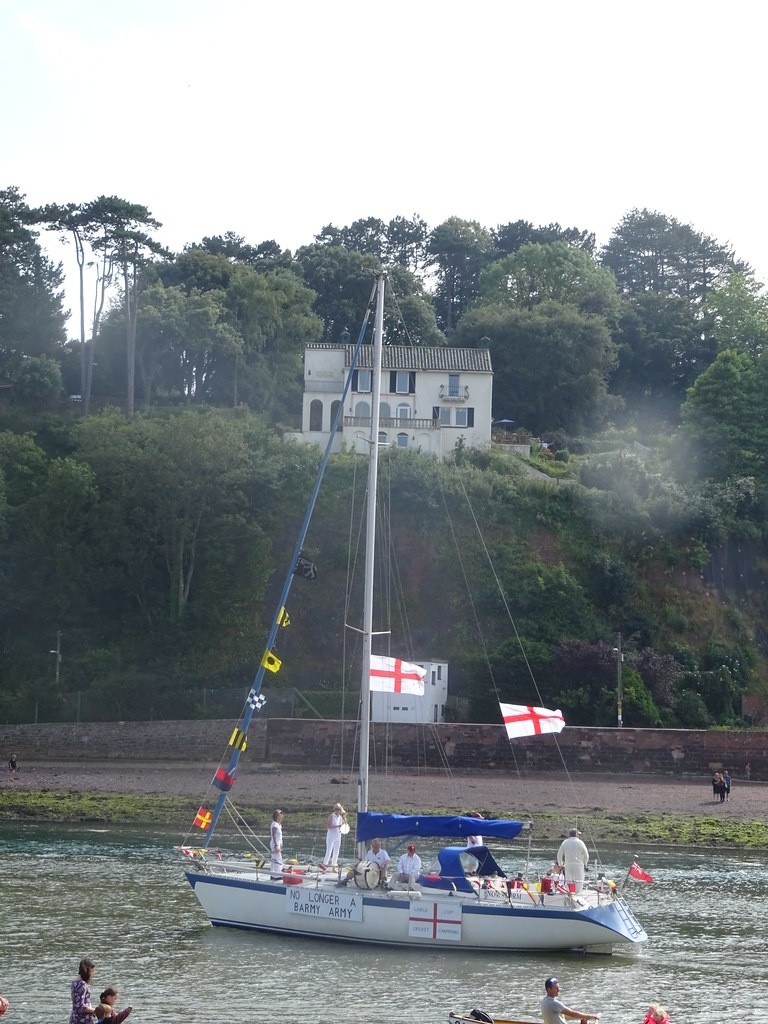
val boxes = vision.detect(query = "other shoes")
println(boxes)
[320,869,326,873]
[334,882,347,887]
[386,888,393,891]
[270,876,283,880]
[333,867,338,873]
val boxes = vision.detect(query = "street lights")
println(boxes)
[612,632,622,728]
[50,630,62,687]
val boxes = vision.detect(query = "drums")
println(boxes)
[354,861,381,889]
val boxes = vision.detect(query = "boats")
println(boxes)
[448,1009,544,1024]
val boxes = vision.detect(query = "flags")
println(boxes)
[261,649,281,674]
[228,729,248,755]
[212,768,233,791]
[629,861,654,883]
[193,808,213,830]
[247,689,267,713]
[500,701,565,739]
[369,654,427,695]
[292,555,318,583]
[276,606,292,631]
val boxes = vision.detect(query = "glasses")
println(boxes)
[548,978,557,984]
[336,809,341,811]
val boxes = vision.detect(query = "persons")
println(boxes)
[334,838,391,889]
[712,770,731,803]
[385,842,422,892]
[465,812,483,847]
[641,1002,671,1024]
[744,762,751,779]
[556,828,589,896]
[69,958,132,1024]
[541,977,599,1024]
[318,802,347,874]
[270,809,283,880]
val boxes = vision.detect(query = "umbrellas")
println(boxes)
[493,418,515,436]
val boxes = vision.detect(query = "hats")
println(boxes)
[570,828,581,834]
[408,846,415,853]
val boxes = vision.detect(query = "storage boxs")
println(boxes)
[282,869,304,884]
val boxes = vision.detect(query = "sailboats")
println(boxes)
[169,270,648,957]
